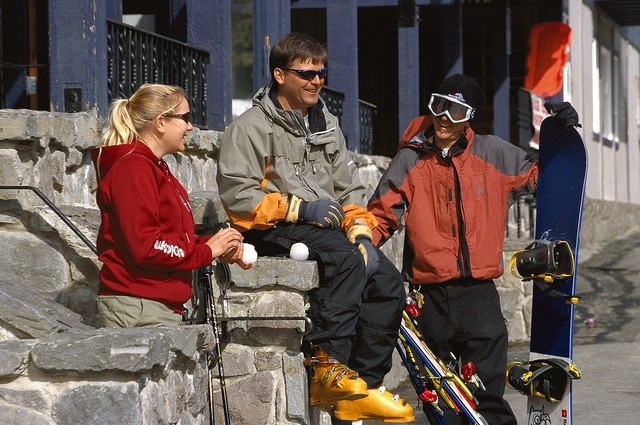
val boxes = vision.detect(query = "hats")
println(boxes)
[436,74,487,110]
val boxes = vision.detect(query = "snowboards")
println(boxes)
[506,115,588,425]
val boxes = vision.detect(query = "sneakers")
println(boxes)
[334,388,414,423]
[309,358,367,405]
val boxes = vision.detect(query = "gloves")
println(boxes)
[299,199,343,230]
[544,101,579,129]
[354,238,379,279]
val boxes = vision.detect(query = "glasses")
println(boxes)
[428,94,475,124]
[165,111,190,123]
[283,68,327,81]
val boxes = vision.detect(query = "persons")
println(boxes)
[216,33,416,424]
[89,83,255,330]
[365,73,580,425]
